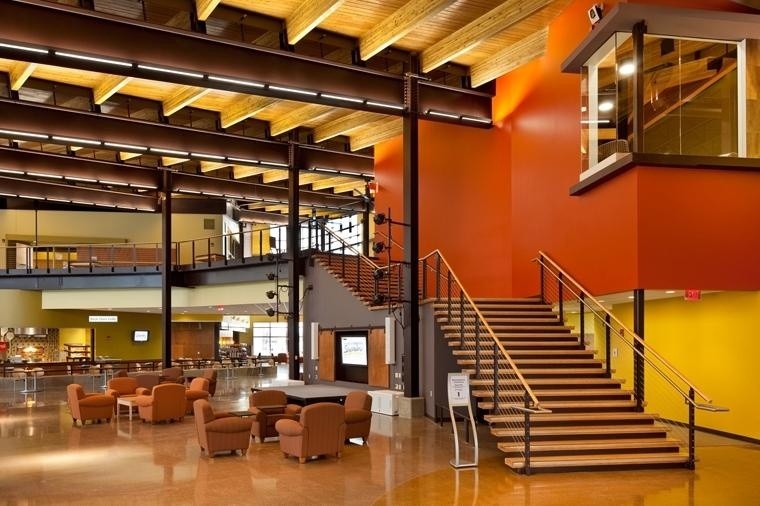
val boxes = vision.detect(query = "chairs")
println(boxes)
[249,390,302,442]
[193,397,252,458]
[343,392,372,442]
[67,367,219,427]
[0,354,289,394]
[274,401,346,464]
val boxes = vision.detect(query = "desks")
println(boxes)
[253,384,356,406]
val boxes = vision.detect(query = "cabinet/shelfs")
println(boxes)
[63,342,90,360]
[368,389,404,416]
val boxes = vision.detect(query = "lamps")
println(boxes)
[371,212,389,304]
[265,252,277,316]
